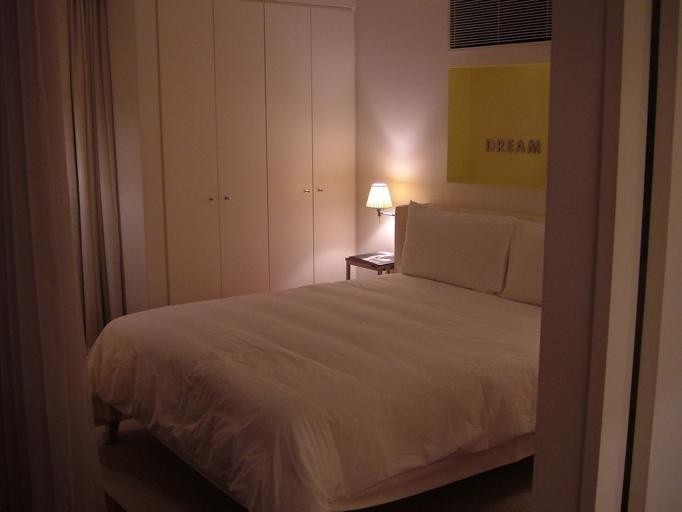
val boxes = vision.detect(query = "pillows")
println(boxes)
[497,218,544,309]
[400,198,515,295]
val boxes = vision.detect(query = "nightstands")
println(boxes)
[344,252,395,280]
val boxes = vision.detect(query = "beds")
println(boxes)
[87,195,542,511]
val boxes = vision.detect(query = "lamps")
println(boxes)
[365,182,395,216]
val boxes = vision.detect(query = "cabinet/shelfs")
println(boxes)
[156,0,268,304]
[265,0,357,291]
